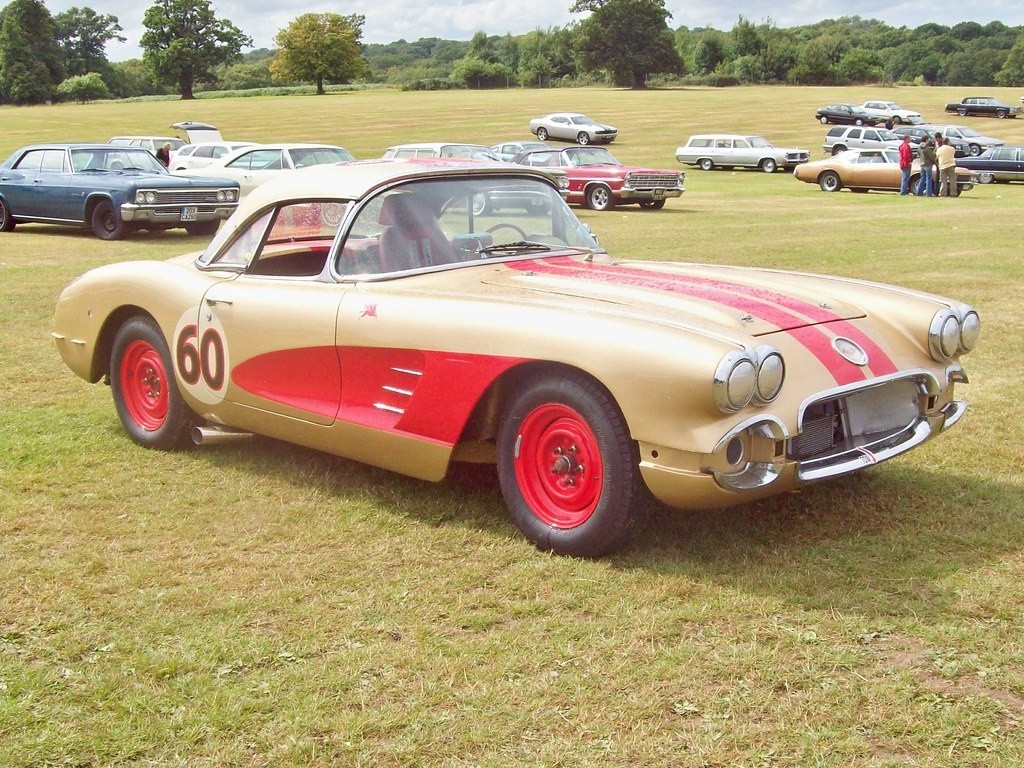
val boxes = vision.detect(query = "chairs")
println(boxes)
[379,193,458,274]
[111,160,124,169]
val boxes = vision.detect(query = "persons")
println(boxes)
[886,116,895,130]
[156,142,171,167]
[897,133,914,196]
[870,153,882,163]
[917,132,957,197]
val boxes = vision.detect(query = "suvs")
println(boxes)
[676,134,810,173]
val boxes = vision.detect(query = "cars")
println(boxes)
[170,122,277,171]
[105,136,188,168]
[814,103,882,126]
[377,140,686,215]
[529,113,618,146]
[0,144,241,241]
[169,145,359,202]
[945,96,1019,119]
[857,100,924,125]
[792,124,1024,197]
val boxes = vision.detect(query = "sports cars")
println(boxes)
[53,160,980,563]
